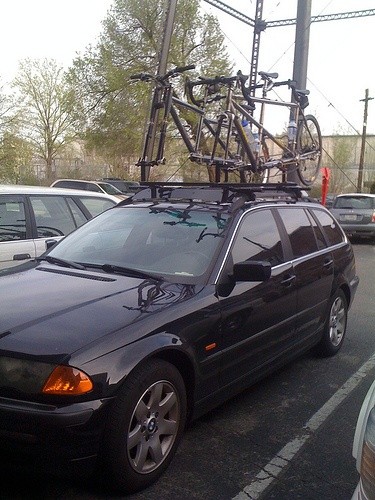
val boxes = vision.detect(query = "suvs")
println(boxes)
[0,184,360,495]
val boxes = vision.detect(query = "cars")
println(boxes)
[330,193,375,239]
[49,178,139,200]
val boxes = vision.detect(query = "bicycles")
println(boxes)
[129,63,323,186]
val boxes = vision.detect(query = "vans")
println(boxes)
[0,183,150,269]
